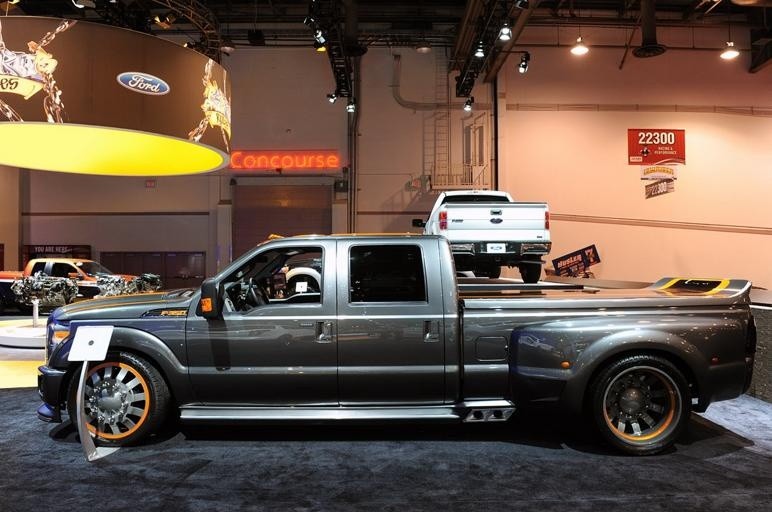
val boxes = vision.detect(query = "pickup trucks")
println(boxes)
[0,257,163,316]
[412,188,553,284]
[35,230,759,457]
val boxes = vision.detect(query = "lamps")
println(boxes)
[460,19,531,113]
[220,22,236,54]
[325,78,358,115]
